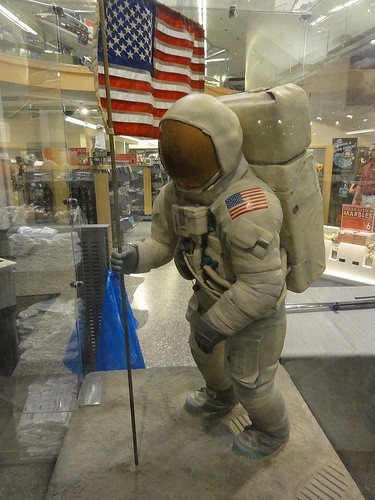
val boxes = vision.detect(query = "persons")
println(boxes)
[15,156,26,187]
[112,93,290,458]
[349,144,375,206]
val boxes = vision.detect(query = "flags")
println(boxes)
[94,0,206,139]
